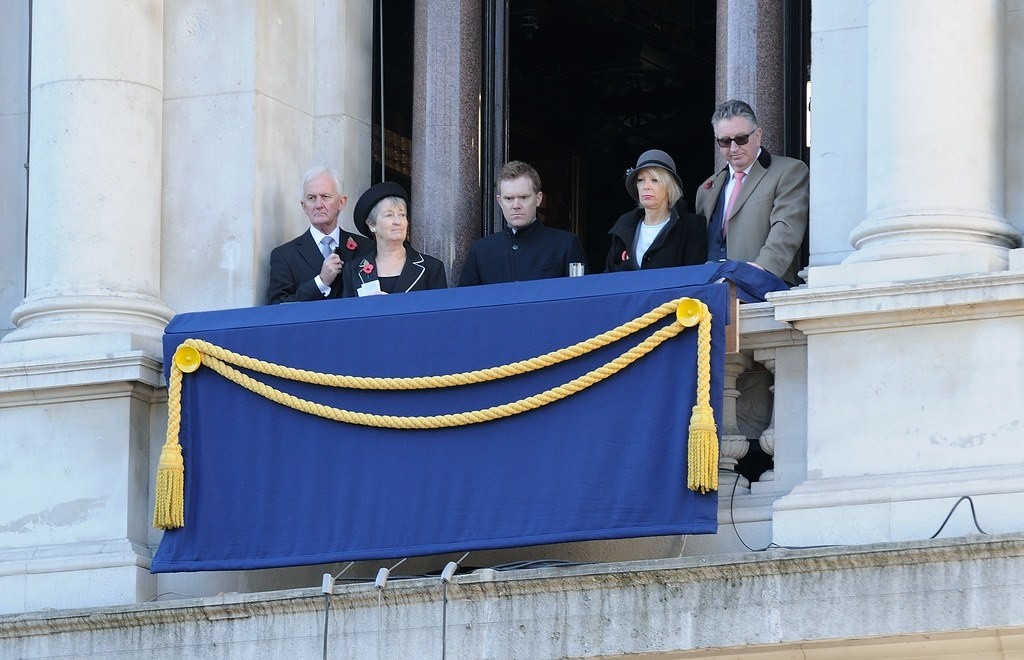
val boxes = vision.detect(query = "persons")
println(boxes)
[695,102,809,481]
[458,161,589,287]
[604,150,708,274]
[266,167,376,305]
[342,183,447,298]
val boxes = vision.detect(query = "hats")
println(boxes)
[626,150,683,199]
[353,182,408,240]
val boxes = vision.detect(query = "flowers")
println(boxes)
[363,259,374,278]
[346,236,358,251]
[705,179,715,189]
[621,250,630,261]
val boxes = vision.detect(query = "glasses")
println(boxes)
[714,132,751,147]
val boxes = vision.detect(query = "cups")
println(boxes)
[569,262,584,277]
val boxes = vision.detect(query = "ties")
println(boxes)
[320,236,334,258]
[722,172,745,238]
